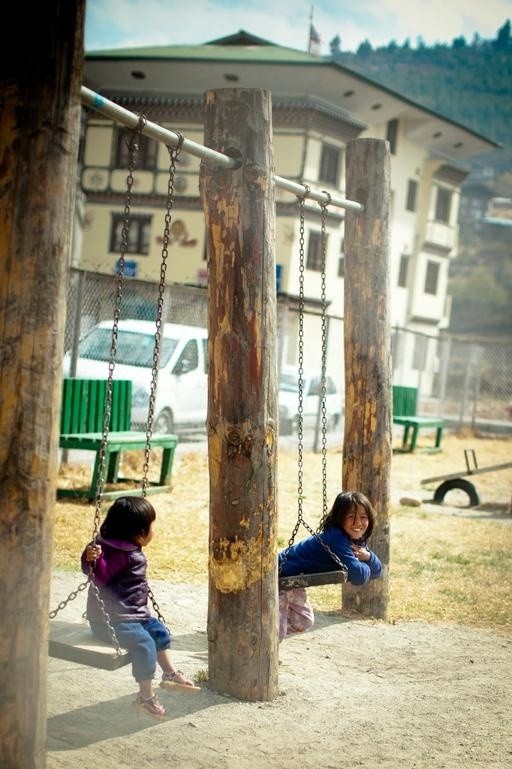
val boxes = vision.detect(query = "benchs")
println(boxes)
[55,378,179,502]
[392,385,444,455]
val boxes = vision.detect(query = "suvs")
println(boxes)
[63,320,209,434]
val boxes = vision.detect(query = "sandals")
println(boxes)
[160,670,201,694]
[134,691,166,720]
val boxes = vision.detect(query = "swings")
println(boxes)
[278,198,347,590]
[48,126,180,670]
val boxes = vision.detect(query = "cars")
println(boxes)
[278,366,345,428]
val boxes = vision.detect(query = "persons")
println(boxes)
[276,489,383,644]
[81,496,204,718]
